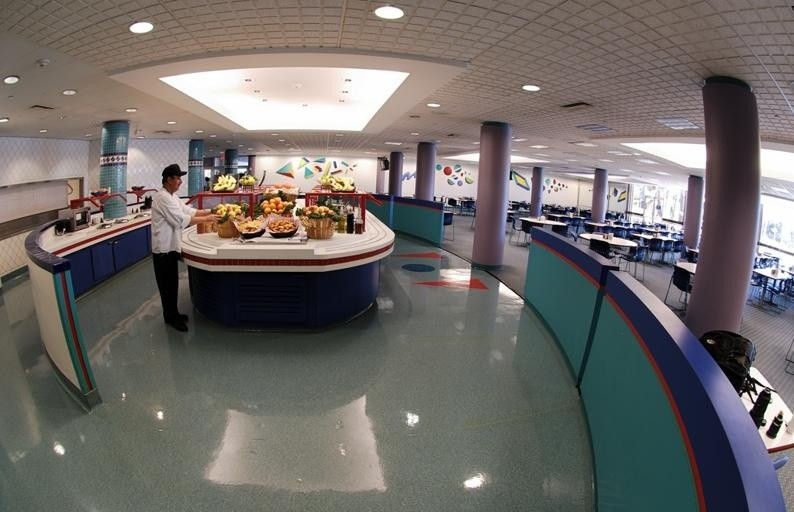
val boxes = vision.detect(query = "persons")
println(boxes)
[150,162,225,333]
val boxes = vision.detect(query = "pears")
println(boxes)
[239,177,256,186]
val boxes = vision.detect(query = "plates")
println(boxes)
[239,226,300,239]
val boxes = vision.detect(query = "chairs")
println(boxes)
[504,199,792,312]
[785,338,794,375]
[435,194,479,241]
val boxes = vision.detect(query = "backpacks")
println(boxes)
[697,330,757,399]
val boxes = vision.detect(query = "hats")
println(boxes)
[162,164,187,176]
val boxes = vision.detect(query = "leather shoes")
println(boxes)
[164,309,189,332]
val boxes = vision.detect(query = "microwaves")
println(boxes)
[58,207,90,231]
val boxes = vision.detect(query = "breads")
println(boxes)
[269,219,296,232]
[240,219,261,232]
[275,182,296,189]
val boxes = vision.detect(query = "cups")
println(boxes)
[607,233,614,241]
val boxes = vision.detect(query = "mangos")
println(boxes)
[217,203,242,218]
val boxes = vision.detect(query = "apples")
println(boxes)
[304,205,335,215]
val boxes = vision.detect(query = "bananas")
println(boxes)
[213,174,237,191]
[322,176,355,191]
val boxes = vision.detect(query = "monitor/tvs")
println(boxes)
[381,160,390,171]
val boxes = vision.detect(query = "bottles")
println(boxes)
[751,387,793,438]
[337,205,363,235]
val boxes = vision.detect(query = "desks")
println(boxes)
[741,367,794,452]
